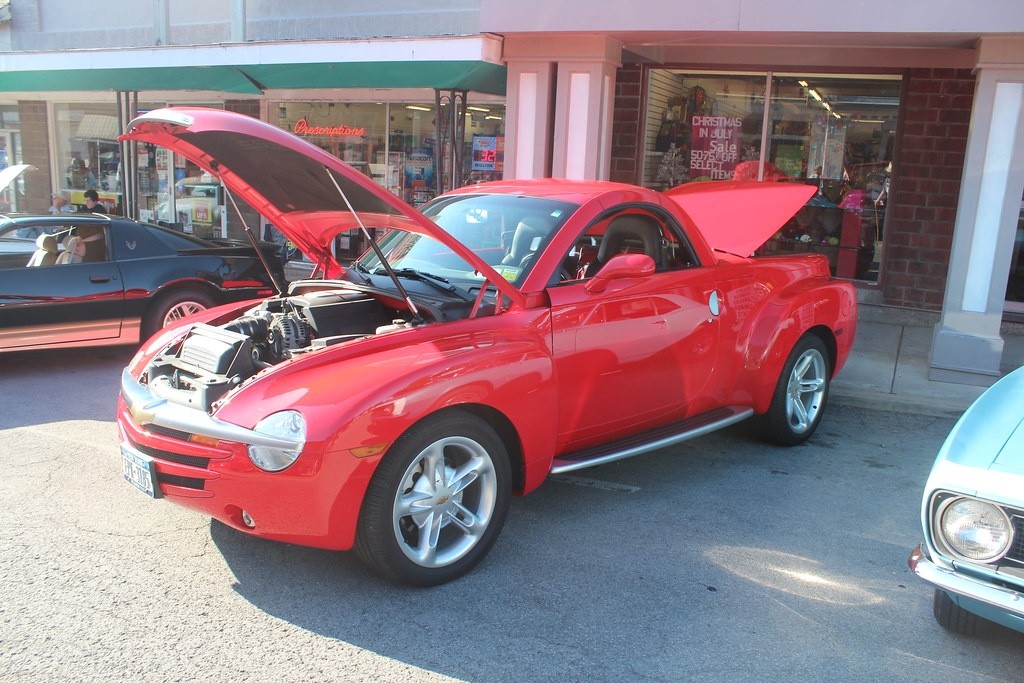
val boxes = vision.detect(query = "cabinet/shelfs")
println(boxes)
[757,178,879,278]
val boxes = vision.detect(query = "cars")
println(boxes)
[0,164,286,354]
[149,177,223,236]
[909,365,1024,639]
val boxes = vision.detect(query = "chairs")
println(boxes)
[55,235,86,265]
[496,212,573,280]
[582,214,666,278]
[25,233,58,268]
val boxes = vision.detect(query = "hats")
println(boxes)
[885,162,892,173]
[50,191,69,201]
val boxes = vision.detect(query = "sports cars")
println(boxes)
[117,107,857,592]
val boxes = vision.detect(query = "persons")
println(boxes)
[808,164,826,178]
[46,189,76,212]
[77,190,108,213]
[873,162,892,207]
[68,156,117,189]
